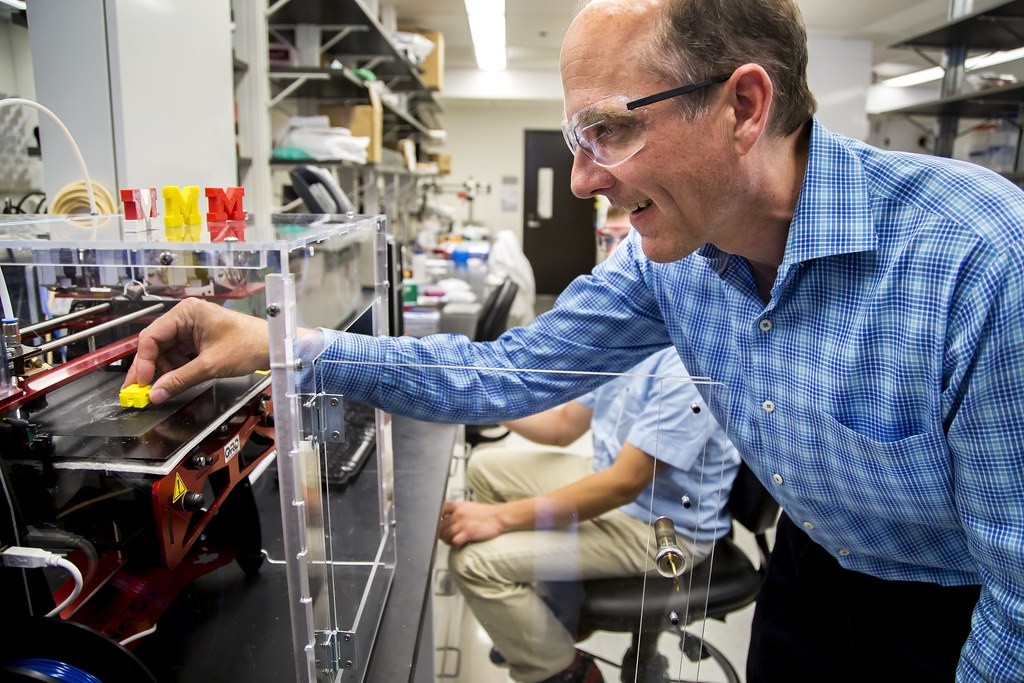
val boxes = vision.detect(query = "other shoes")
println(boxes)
[489,646,506,665]
[549,658,604,683]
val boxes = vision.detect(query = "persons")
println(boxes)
[119,0,1024,683]
[437,204,742,683]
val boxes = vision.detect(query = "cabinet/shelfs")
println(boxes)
[232,0,443,215]
[884,0,1024,186]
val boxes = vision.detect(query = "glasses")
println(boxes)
[560,74,731,167]
[598,235,625,252]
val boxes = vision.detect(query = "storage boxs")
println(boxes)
[320,79,384,167]
[396,28,443,90]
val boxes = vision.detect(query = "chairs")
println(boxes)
[531,462,780,683]
[462,279,516,449]
